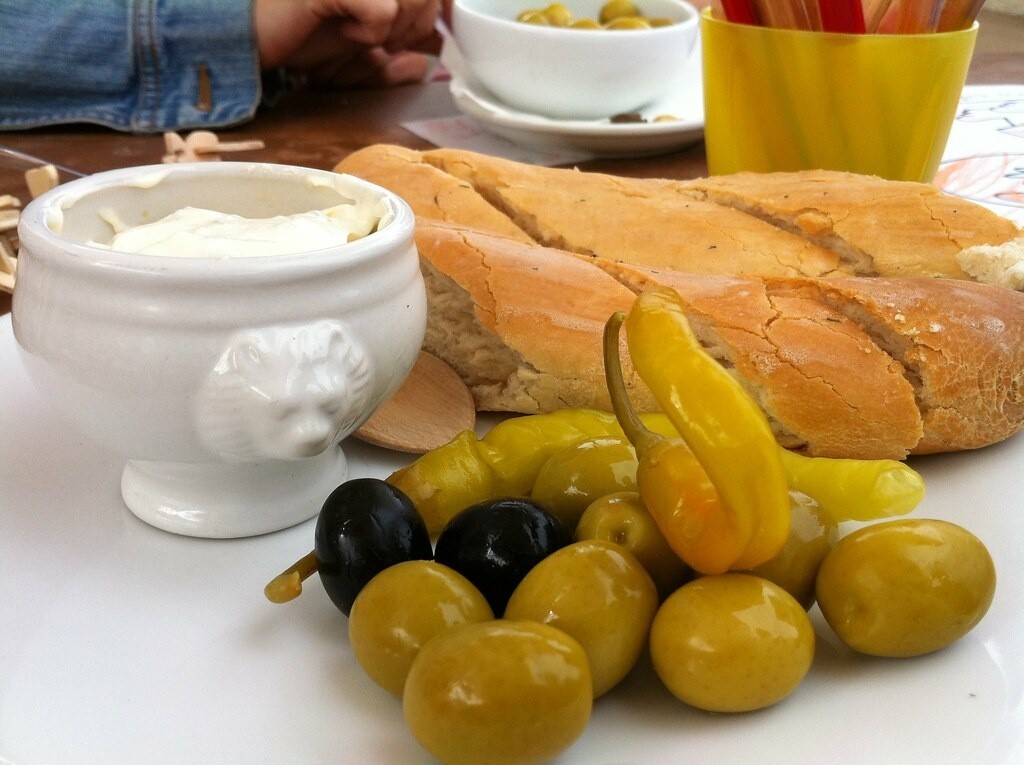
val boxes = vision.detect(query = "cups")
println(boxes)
[701,6,979,185]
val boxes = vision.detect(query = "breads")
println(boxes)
[329,144,1024,460]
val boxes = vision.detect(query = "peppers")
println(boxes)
[601,286,792,576]
[264,409,926,605]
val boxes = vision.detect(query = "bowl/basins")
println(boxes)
[450,0,699,116]
[12,158,429,540]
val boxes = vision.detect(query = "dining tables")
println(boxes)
[0,50,1024,335]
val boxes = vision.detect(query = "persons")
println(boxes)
[0,0,454,135]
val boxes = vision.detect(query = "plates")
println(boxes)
[0,309,1023,765]
[450,46,704,154]
